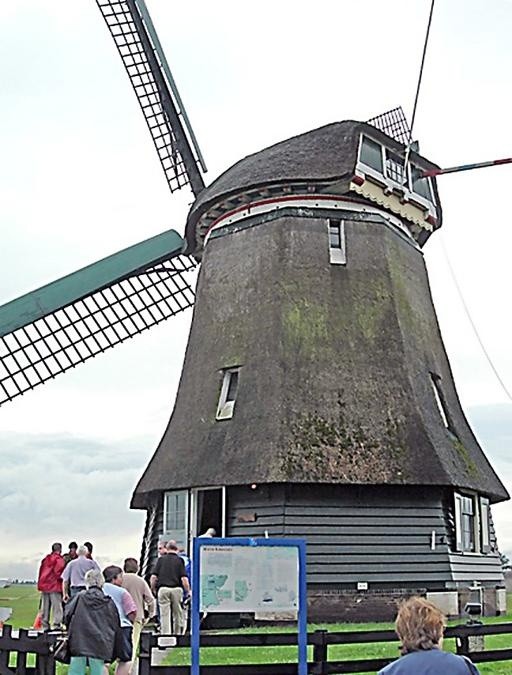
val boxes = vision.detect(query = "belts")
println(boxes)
[72,586,86,589]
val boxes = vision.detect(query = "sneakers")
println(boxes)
[52,623,67,630]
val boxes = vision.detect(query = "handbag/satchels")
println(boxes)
[53,639,71,663]
[34,596,43,628]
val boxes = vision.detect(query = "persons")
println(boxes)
[37,527,216,675]
[375,595,481,675]
